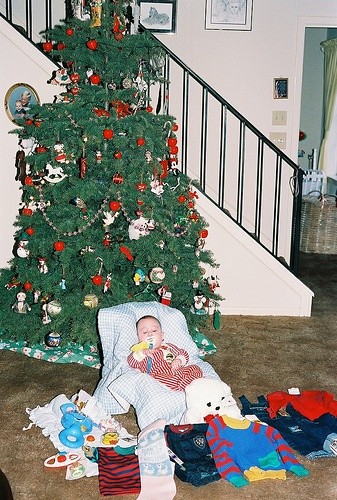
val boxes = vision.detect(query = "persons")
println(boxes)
[14,100,26,116]
[21,91,32,107]
[127,315,203,392]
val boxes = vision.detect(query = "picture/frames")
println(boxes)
[136,0,176,33]
[204,0,253,31]
[274,78,289,100]
[5,83,42,127]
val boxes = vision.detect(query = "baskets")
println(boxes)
[299,188,337,256]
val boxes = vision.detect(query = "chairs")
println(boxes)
[97,301,231,431]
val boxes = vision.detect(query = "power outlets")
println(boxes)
[272,111,287,125]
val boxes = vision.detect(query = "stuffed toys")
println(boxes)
[179,378,230,425]
